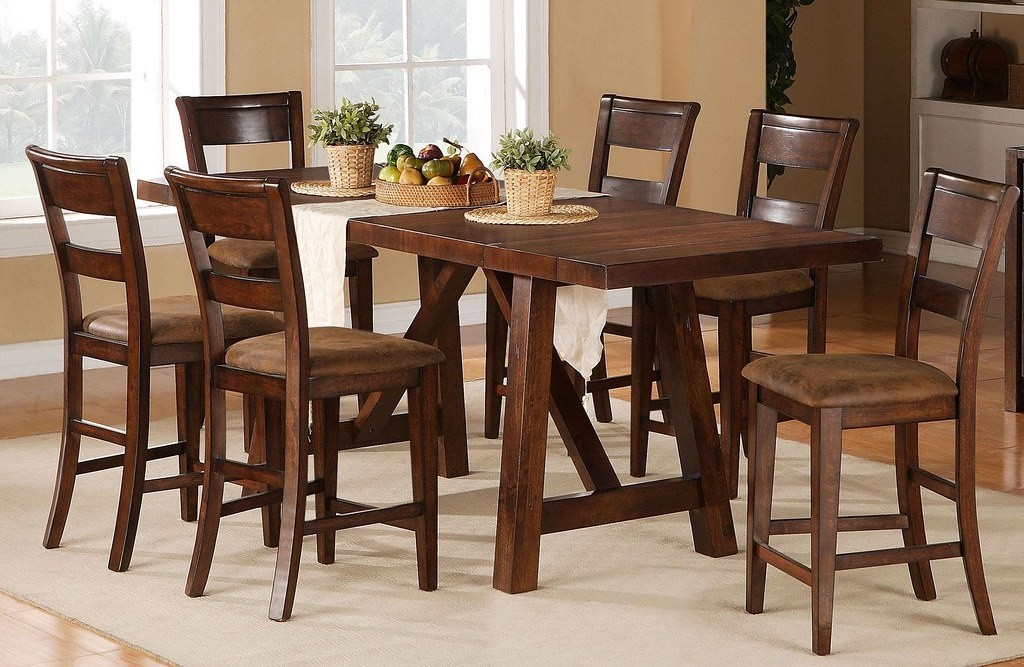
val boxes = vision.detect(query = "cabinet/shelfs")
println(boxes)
[910,0,1024,275]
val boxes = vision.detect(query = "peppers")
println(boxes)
[387,144,413,165]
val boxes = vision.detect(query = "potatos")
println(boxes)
[398,168,423,185]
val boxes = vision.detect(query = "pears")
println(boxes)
[375,162,400,182]
[459,149,485,181]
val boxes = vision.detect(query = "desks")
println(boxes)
[137,165,885,593]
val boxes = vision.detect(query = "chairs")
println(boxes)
[26,143,282,573]
[175,90,379,454]
[485,94,701,440]
[629,109,861,499]
[741,166,1020,657]
[164,166,445,622]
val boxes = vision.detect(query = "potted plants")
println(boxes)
[303,96,395,188]
[487,126,575,215]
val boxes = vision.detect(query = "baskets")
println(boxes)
[322,144,376,190]
[373,162,501,208]
[503,166,558,216]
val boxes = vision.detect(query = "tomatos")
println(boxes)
[396,154,461,179]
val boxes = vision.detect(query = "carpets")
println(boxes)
[0,376,1024,667]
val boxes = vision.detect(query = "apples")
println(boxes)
[455,174,478,185]
[418,143,444,160]
